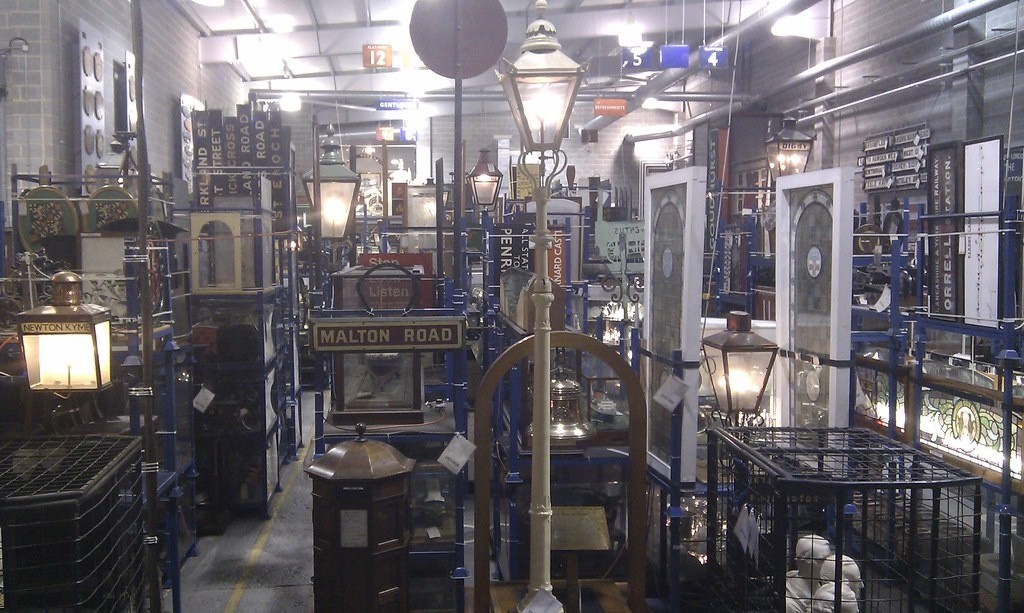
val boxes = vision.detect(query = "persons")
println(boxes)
[784,532,866,613]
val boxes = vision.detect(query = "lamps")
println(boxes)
[16,270,115,400]
[701,310,780,416]
[192,0,225,7]
[301,121,362,243]
[765,116,818,183]
[492,0,587,153]
[263,13,298,35]
[278,95,301,112]
[465,148,504,212]
[604,21,648,47]
